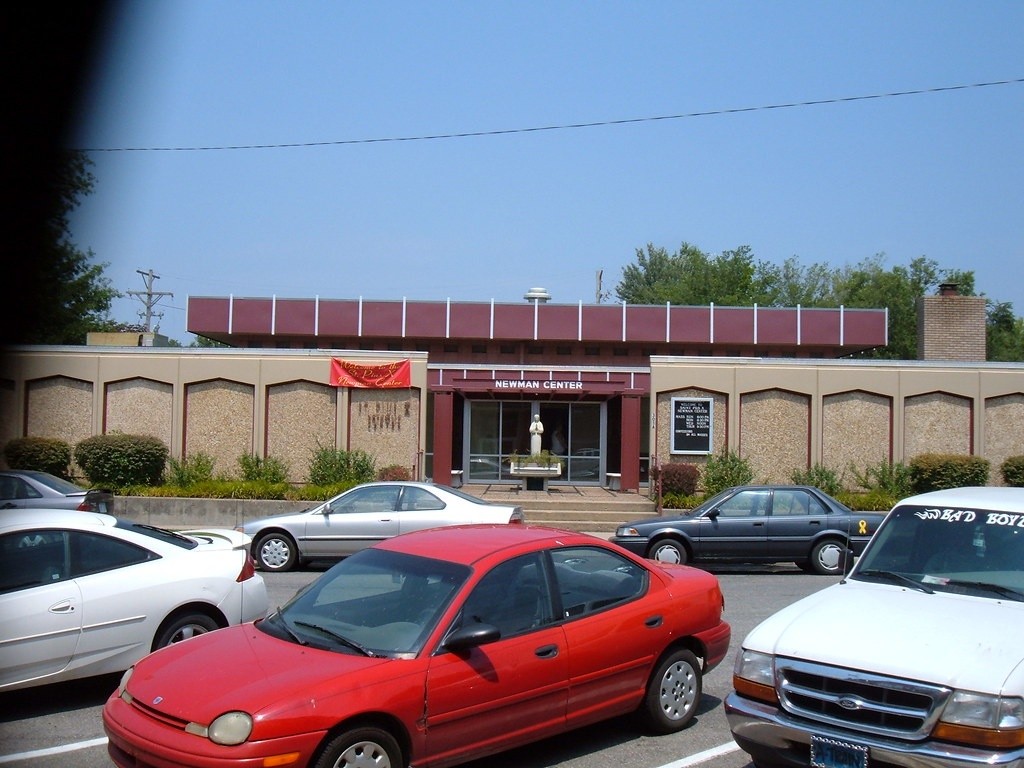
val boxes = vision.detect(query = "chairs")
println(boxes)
[388,569,430,622]
[478,567,546,633]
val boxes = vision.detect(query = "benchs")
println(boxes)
[524,561,637,605]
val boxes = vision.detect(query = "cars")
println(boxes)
[0,509,270,694]
[606,486,921,575]
[0,469,113,517]
[102,524,732,767]
[468,460,511,481]
[233,481,522,573]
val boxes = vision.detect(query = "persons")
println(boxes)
[529,414,544,456]
[551,423,566,455]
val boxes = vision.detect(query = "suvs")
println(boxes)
[571,448,600,470]
[723,487,1024,768]
